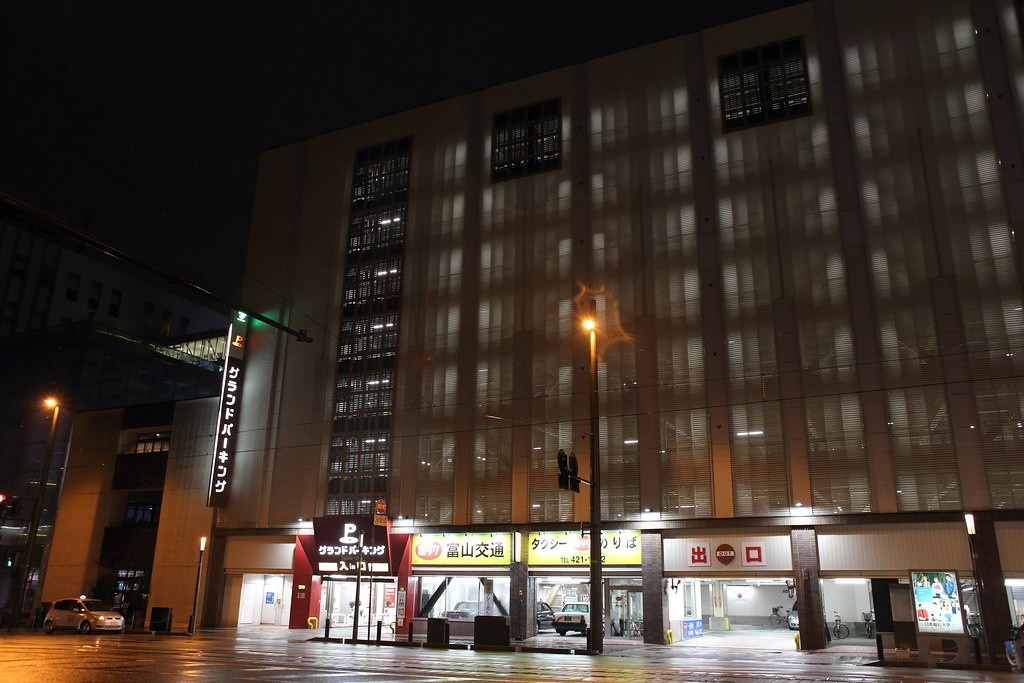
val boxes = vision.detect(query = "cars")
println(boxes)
[786,599,801,629]
[535,600,555,635]
[43,598,127,633]
[442,600,484,620]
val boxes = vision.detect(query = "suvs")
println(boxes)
[550,601,607,637]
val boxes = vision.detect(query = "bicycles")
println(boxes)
[768,606,790,627]
[832,611,850,639]
[861,610,875,639]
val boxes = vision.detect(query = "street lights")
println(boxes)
[351,529,365,644]
[581,297,607,653]
[963,513,996,668]
[186,536,208,635]
[10,398,60,629]
